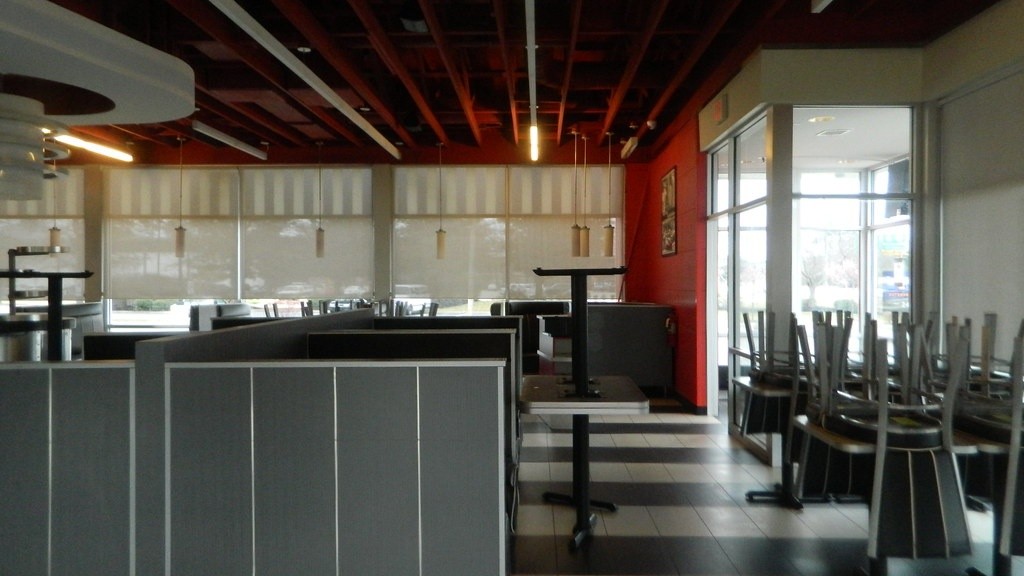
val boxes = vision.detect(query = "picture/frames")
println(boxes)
[659,165,678,257]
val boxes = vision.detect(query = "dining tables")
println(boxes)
[732,365,1023,576]
[515,266,651,409]
[519,402,648,555]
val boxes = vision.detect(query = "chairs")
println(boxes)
[263,297,440,317]
[740,308,1023,558]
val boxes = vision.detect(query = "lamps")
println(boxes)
[568,128,639,259]
[173,137,188,258]
[435,141,446,260]
[49,157,62,259]
[314,140,326,259]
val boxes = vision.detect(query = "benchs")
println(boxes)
[490,301,570,374]
[587,303,676,396]
[189,303,251,334]
